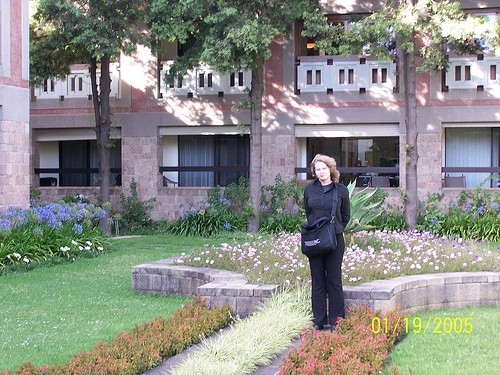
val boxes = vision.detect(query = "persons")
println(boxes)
[300,154,350,332]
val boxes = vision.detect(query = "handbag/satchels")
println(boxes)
[301,217,337,255]
